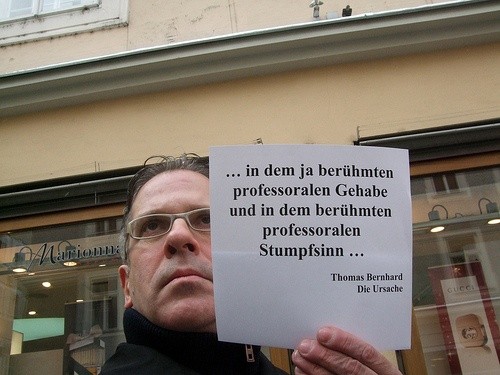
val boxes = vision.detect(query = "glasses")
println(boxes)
[122,206,212,242]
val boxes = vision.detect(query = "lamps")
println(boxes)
[56,239,79,268]
[427,204,450,234]
[13,246,33,273]
[478,196,500,227]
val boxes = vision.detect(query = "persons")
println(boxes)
[457,314,499,375]
[84,155,405,375]
